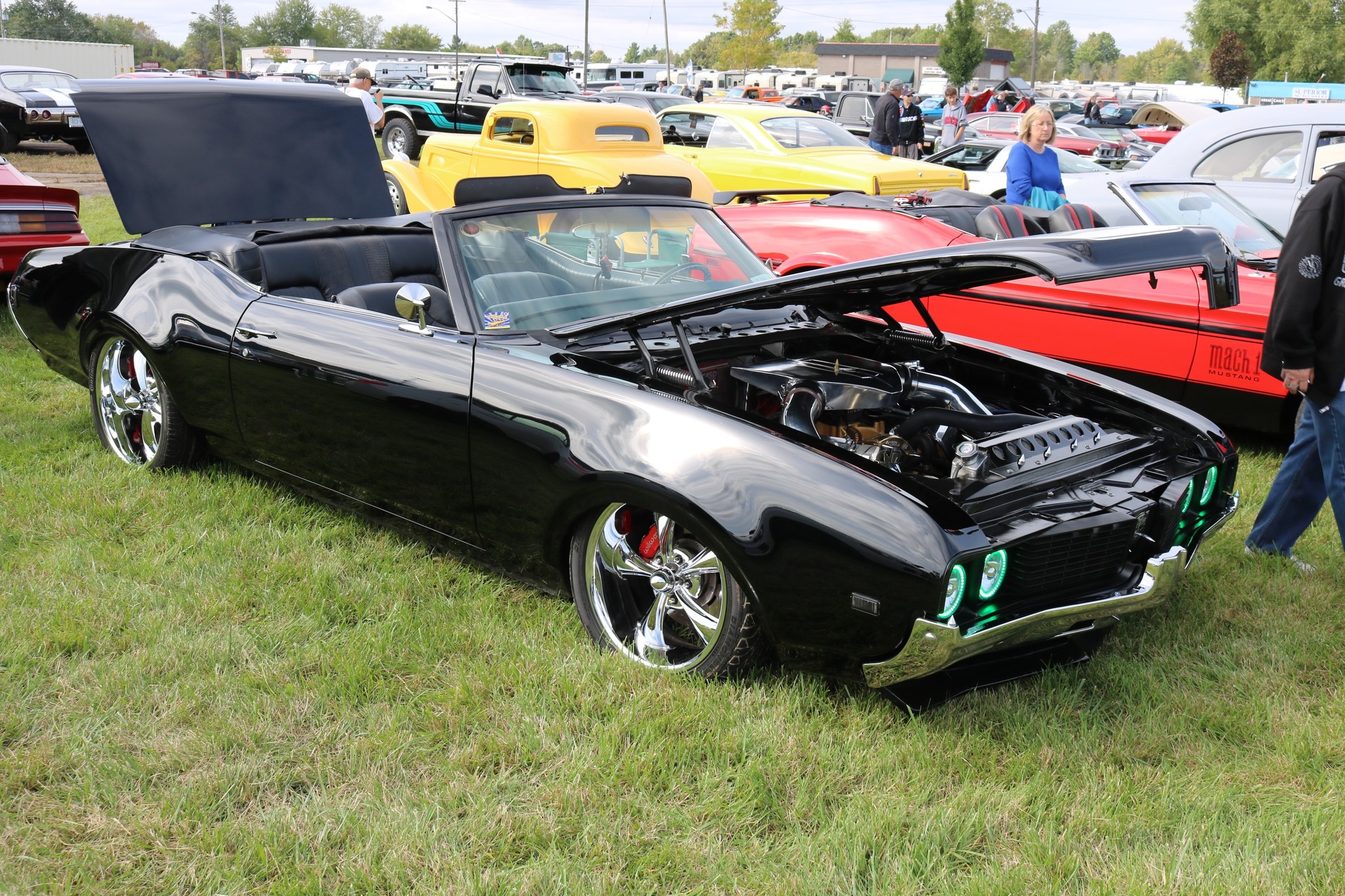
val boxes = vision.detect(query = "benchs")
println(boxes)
[254,224,445,302]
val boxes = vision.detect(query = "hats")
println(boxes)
[1004,90,1008,94]
[901,87,915,96]
[889,80,906,90]
[1096,100,1104,103]
[349,67,378,86]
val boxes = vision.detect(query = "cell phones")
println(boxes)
[370,89,380,95]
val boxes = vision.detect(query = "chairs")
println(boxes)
[331,282,458,330]
[1048,204,1109,233]
[975,204,1047,240]
[473,272,575,311]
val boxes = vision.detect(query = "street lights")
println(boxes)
[426,6,458,79]
[566,45,579,75]
[190,11,227,70]
[1015,8,1041,105]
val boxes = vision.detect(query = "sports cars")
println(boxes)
[685,177,1305,449]
[7,80,1243,716]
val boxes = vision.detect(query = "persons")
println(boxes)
[993,91,1009,112]
[680,83,692,98]
[867,78,903,156]
[1245,161,1345,568]
[1004,103,1067,211]
[694,84,703,103]
[915,96,923,105]
[656,81,667,93]
[337,67,385,140]
[899,88,925,160]
[1083,96,1103,124]
[940,86,969,162]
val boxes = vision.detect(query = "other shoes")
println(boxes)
[1244,545,1317,572]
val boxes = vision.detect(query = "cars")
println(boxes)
[376,99,735,254]
[1,155,91,273]
[1061,102,1345,235]
[651,102,969,206]
[917,137,1114,202]
[109,56,1345,171]
[0,65,95,155]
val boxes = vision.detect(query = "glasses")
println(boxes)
[906,95,913,97]
[902,87,905,90]
[1031,122,1055,128]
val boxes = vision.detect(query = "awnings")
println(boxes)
[881,69,914,83]
